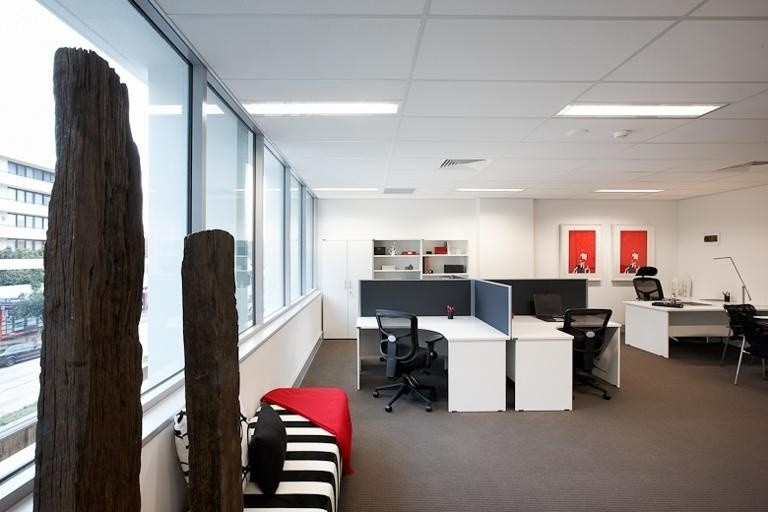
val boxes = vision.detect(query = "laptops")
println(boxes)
[533,293,576,322]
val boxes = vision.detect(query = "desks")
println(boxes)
[355,312,509,415]
[513,311,622,412]
[621,296,767,359]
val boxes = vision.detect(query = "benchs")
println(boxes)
[242,388,349,512]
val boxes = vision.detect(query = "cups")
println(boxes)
[725,296,730,302]
[448,312,453,319]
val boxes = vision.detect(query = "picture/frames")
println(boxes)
[558,224,658,283]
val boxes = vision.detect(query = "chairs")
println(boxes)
[555,307,614,400]
[631,264,666,302]
[719,302,768,385]
[374,307,443,413]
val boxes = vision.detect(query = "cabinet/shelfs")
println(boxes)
[371,237,472,280]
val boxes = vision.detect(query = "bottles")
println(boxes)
[671,293,676,305]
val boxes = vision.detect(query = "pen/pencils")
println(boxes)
[447,305,455,312]
[722,291,731,296]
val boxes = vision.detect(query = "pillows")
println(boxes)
[171,401,287,500]
